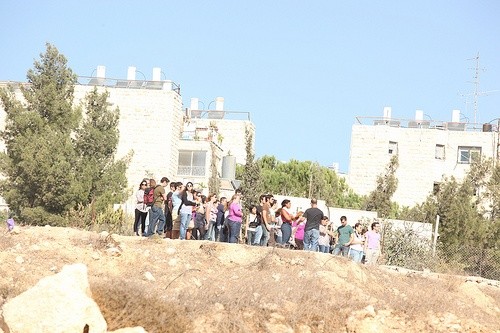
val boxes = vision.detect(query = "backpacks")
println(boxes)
[144,185,162,206]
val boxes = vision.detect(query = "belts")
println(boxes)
[283,222,291,226]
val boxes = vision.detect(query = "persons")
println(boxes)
[244,194,381,266]
[133,176,170,237]
[165,181,243,244]
[6,215,14,233]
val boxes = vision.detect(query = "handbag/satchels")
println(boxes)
[249,222,258,228]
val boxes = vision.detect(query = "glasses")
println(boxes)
[141,184,147,186]
[188,185,192,187]
